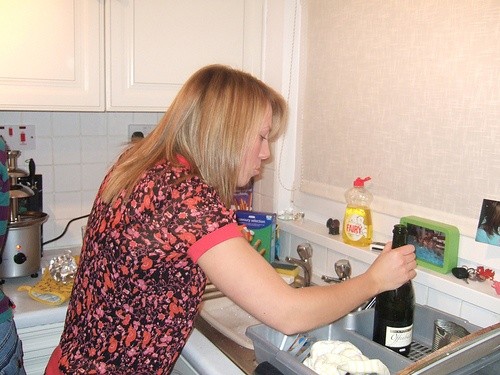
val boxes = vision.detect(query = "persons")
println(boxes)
[0,134,30,375]
[45,64,418,375]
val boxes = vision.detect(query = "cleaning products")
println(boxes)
[339,170,376,245]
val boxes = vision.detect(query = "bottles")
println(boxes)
[372,224,415,356]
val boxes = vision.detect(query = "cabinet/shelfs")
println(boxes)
[0,0,270,114]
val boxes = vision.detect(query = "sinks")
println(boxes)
[197,296,268,348]
[248,322,414,375]
[335,303,490,362]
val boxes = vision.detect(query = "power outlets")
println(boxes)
[128,123,159,145]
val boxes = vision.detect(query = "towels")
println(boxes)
[303,339,391,374]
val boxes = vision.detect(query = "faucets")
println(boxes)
[322,258,352,286]
[283,241,314,289]
